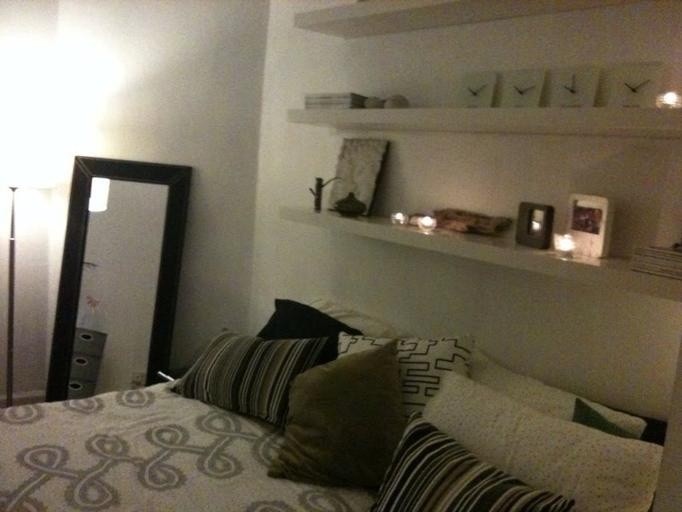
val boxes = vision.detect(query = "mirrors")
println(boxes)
[45,155,193,402]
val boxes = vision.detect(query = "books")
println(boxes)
[629,244,682,281]
[303,91,368,110]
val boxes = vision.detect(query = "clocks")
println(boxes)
[457,62,664,108]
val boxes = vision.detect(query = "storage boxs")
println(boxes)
[66,327,109,398]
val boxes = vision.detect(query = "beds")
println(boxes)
[1,298,669,511]
